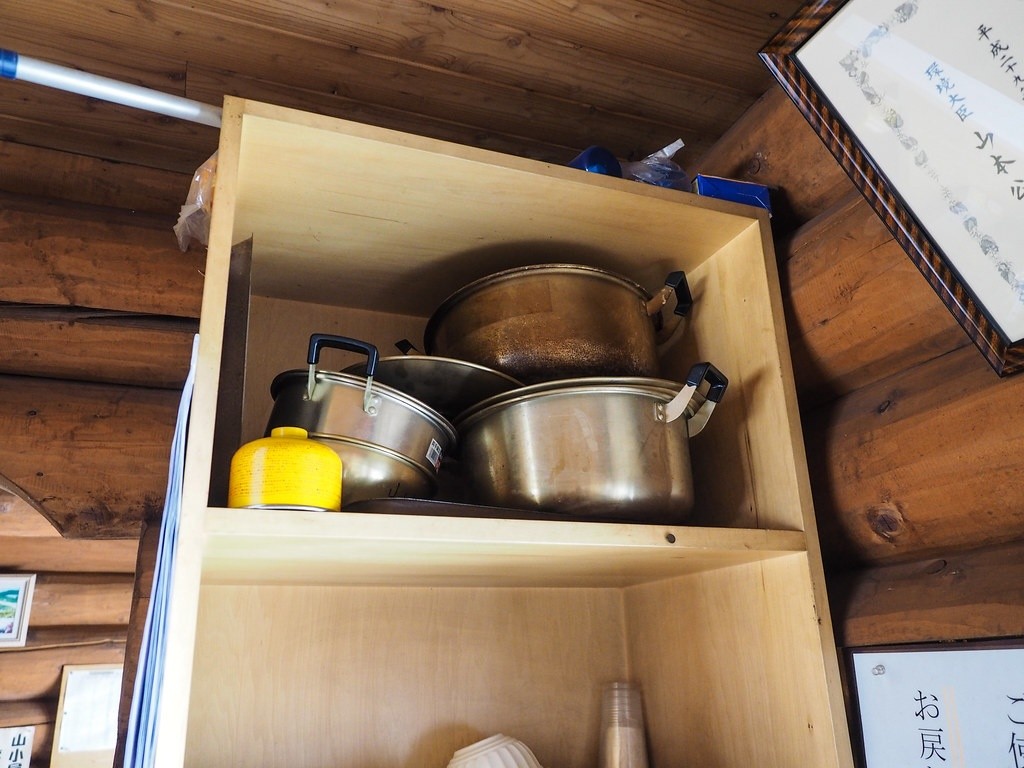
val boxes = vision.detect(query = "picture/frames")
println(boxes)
[760,0,1024,377]
[844,639,1024,768]
[0,573,36,647]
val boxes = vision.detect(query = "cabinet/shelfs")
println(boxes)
[150,94,857,768]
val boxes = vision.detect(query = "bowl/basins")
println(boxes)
[447,734,543,768]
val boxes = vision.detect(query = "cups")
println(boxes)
[600,681,648,768]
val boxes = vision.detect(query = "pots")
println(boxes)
[264,334,457,507]
[454,362,729,517]
[395,263,693,387]
[338,354,524,423]
